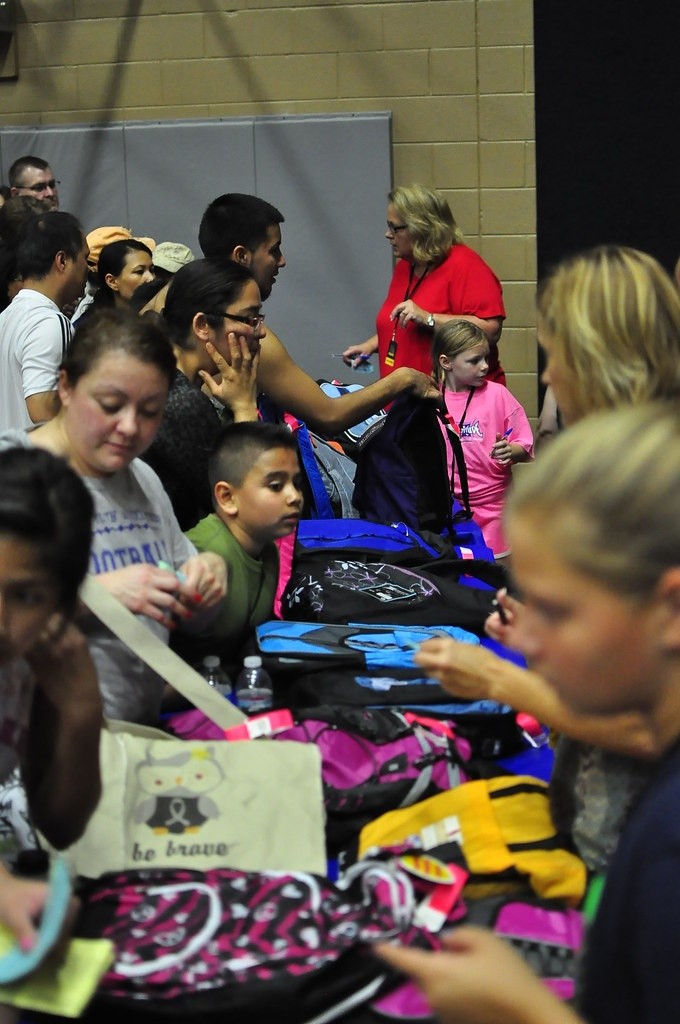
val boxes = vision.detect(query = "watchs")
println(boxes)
[428,313,435,326]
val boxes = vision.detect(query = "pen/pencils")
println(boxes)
[491,599,511,626]
[332,354,371,359]
[490,428,512,455]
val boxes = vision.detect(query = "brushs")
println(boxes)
[385,315,400,368]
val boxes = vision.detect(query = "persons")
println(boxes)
[0,155,680,1024]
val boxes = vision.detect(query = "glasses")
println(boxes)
[200,312,266,331]
[15,180,59,192]
[387,222,407,233]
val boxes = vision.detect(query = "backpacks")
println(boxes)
[273,559,517,637]
[292,518,457,571]
[315,378,389,463]
[159,708,472,872]
[351,389,474,535]
[245,620,531,762]
[289,421,363,519]
[71,868,415,1024]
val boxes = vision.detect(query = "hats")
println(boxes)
[152,242,195,273]
[85,226,156,273]
